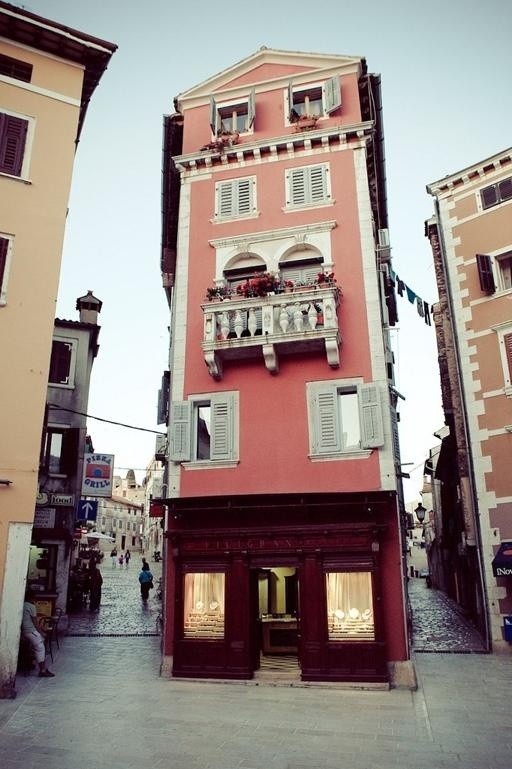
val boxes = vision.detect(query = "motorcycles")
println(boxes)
[152,551,160,562]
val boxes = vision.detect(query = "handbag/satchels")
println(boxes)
[150,582,153,589]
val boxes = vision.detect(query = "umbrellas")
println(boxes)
[85,532,115,550]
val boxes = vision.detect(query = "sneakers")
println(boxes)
[24,663,55,677]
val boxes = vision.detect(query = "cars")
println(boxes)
[420,568,429,578]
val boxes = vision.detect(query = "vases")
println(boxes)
[298,119,316,128]
[222,134,238,142]
[211,296,221,303]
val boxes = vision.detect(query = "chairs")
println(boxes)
[36,607,62,662]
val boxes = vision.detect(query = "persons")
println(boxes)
[118,554,124,569]
[111,547,117,569]
[138,566,153,601]
[20,589,56,678]
[82,546,104,612]
[124,550,131,567]
[140,557,150,599]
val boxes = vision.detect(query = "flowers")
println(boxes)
[216,129,239,135]
[301,114,319,120]
[206,271,337,300]
